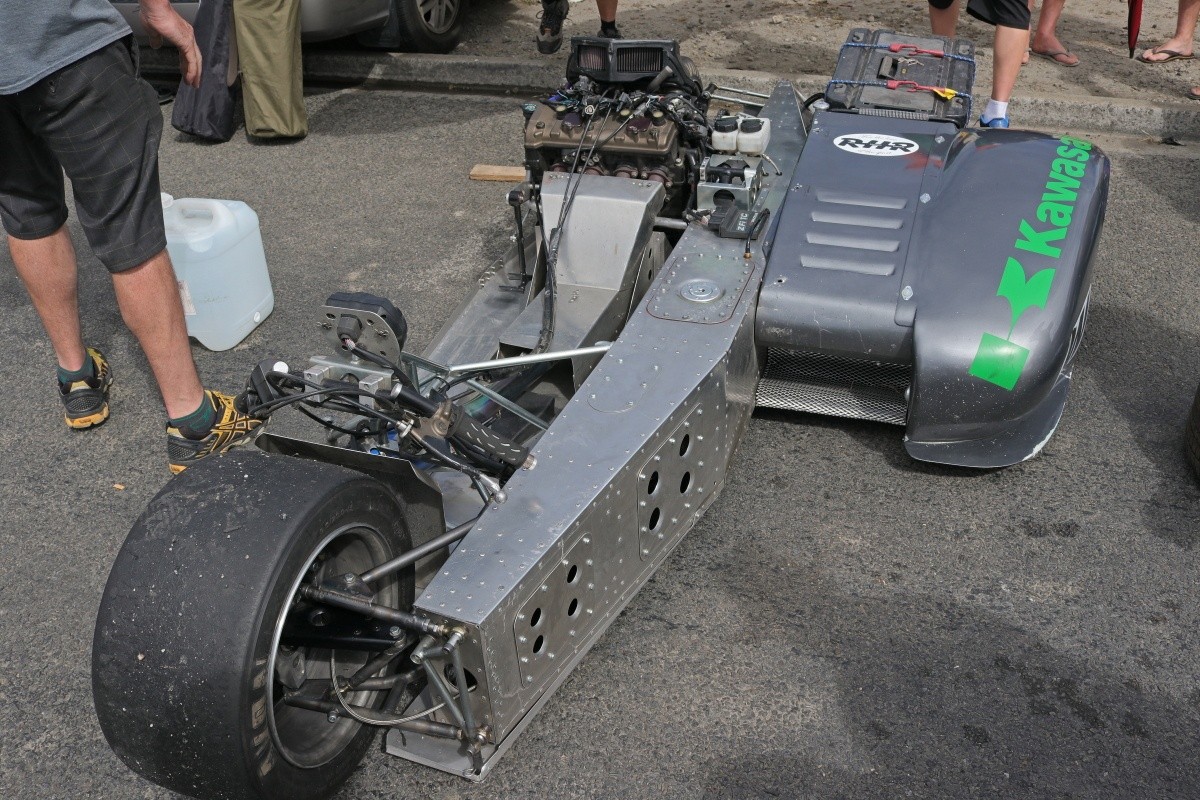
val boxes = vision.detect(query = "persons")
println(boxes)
[1020,0,1080,66]
[928,0,1031,129]
[1136,0,1200,100]
[536,0,625,54]
[0,0,273,477]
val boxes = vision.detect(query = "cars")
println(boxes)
[106,0,473,76]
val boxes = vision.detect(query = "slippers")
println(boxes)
[1030,48,1080,67]
[1021,48,1033,66]
[1138,46,1197,63]
[1188,86,1200,100]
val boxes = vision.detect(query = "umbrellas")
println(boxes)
[1128,0,1143,58]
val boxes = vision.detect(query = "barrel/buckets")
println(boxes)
[160,191,275,352]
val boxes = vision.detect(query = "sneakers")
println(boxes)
[58,347,113,428]
[597,28,624,40]
[535,0,572,54]
[166,390,272,477]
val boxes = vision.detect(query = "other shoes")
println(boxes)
[980,114,1009,129]
